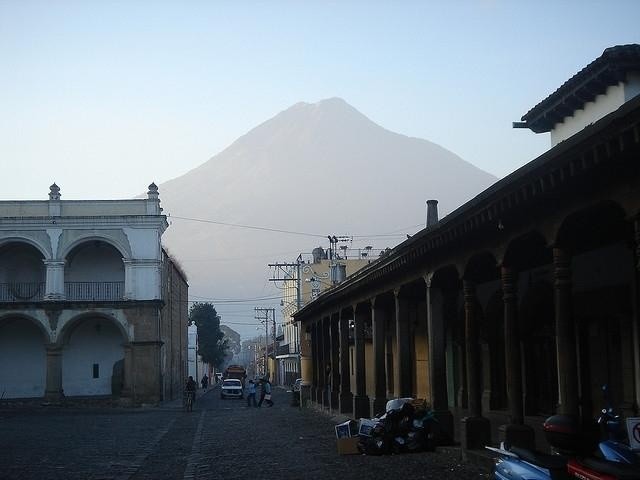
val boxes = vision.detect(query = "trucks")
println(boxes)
[223,365,247,388]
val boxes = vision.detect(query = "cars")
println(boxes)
[221,378,244,400]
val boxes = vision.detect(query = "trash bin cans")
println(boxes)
[287,391,299,407]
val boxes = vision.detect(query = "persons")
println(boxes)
[247,379,258,409]
[215,374,219,386]
[200,373,209,392]
[265,380,271,395]
[258,379,274,408]
[184,375,197,412]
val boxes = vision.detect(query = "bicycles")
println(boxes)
[182,390,194,412]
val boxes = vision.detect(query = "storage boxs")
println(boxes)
[335,418,375,455]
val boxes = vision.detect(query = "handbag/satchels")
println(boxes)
[264,393,271,401]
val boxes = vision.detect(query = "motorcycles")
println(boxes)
[485,383,640,480]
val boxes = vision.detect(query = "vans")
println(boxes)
[216,373,223,381]
